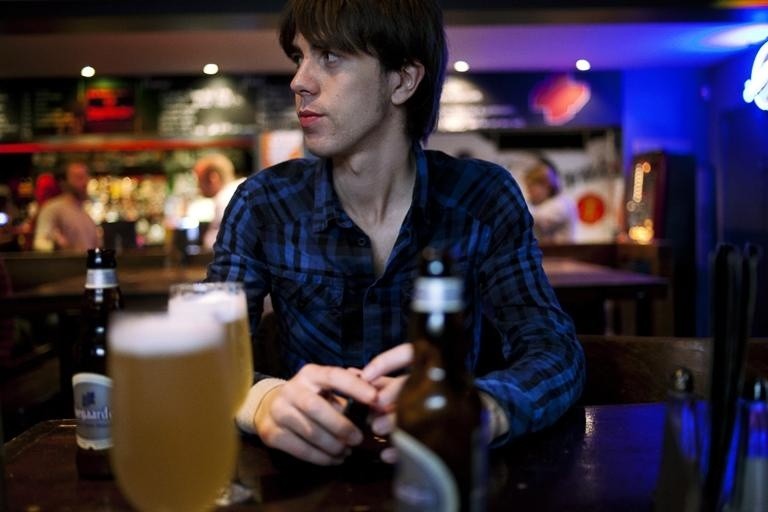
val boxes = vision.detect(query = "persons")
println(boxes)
[195,0,588,468]
[195,152,249,252]
[30,154,101,252]
[521,156,587,247]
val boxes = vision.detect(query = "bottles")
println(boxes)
[389,238,486,512]
[648,360,768,512]
[71,248,131,483]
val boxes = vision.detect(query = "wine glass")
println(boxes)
[168,281,256,509]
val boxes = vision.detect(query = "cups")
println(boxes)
[106,312,240,512]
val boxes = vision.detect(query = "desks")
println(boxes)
[1,255,672,418]
[0,396,767,512]
